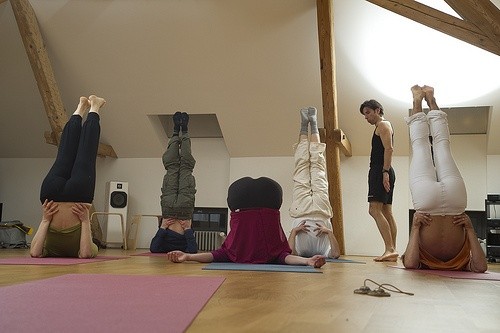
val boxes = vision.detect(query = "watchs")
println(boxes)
[382,169,389,172]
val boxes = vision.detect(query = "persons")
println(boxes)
[359,98,399,263]
[167,176,326,269]
[289,105,341,259]
[148,111,198,255]
[401,84,488,273]
[29,95,106,258]
